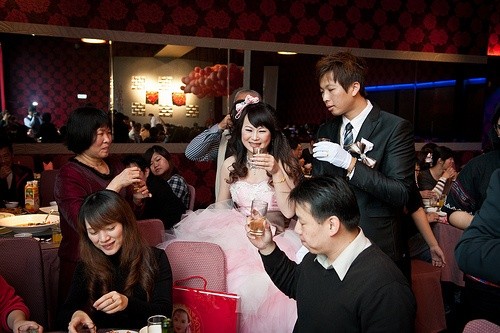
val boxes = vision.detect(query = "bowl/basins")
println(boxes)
[436,212,447,216]
[5,202,19,208]
[425,206,438,212]
[0,214,60,234]
[50,201,57,205]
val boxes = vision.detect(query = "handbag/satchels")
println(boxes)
[172,276,241,333]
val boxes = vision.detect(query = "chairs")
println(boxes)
[0,237,48,333]
[411,259,447,333]
[165,241,227,292]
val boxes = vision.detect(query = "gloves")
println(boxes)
[312,140,352,170]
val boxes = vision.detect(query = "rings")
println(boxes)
[111,298,115,302]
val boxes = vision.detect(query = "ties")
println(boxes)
[344,123,354,157]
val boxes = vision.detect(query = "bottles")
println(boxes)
[162,318,174,333]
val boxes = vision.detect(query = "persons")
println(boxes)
[280,122,320,173]
[311,51,416,285]
[185,88,263,172]
[0,105,65,143]
[246,173,415,333]
[0,274,43,333]
[0,138,37,206]
[127,145,191,209]
[55,107,154,324]
[157,96,306,333]
[56,190,173,333]
[107,109,225,143]
[408,98,500,333]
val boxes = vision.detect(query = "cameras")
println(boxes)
[34,111,40,117]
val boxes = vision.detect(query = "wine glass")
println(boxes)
[437,193,444,212]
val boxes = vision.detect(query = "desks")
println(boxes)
[0,206,62,311]
[430,222,465,286]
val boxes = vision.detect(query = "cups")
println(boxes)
[127,163,141,187]
[53,224,63,247]
[18,324,39,333]
[14,233,32,238]
[147,315,167,333]
[251,200,270,234]
[252,147,267,162]
[76,322,96,333]
[430,195,437,206]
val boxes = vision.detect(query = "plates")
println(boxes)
[0,212,16,234]
[139,325,162,333]
[38,205,58,214]
[106,329,138,333]
